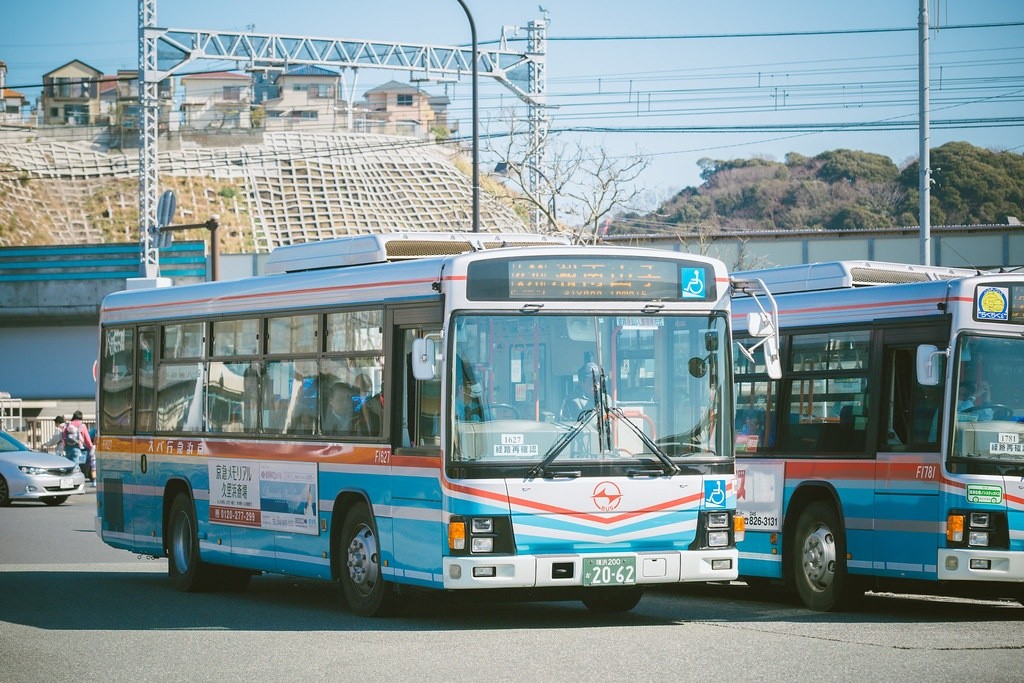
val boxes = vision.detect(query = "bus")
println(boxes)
[620,258,1024,613]
[93,228,783,615]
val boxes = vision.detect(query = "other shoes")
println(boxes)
[90,481,95,487]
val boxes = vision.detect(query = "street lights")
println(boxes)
[494,161,557,221]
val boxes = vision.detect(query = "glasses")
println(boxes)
[465,380,479,385]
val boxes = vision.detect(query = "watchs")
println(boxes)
[967,395,976,401]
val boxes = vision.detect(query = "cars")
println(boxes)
[0,429,86,507]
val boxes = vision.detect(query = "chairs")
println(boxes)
[911,393,939,446]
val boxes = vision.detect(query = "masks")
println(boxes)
[463,383,482,397]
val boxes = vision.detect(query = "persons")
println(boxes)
[559,362,611,422]
[293,372,383,437]
[42,410,96,488]
[928,380,993,443]
[431,367,491,437]
[735,411,774,448]
[222,413,245,433]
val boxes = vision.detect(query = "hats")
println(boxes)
[54,416,64,423]
